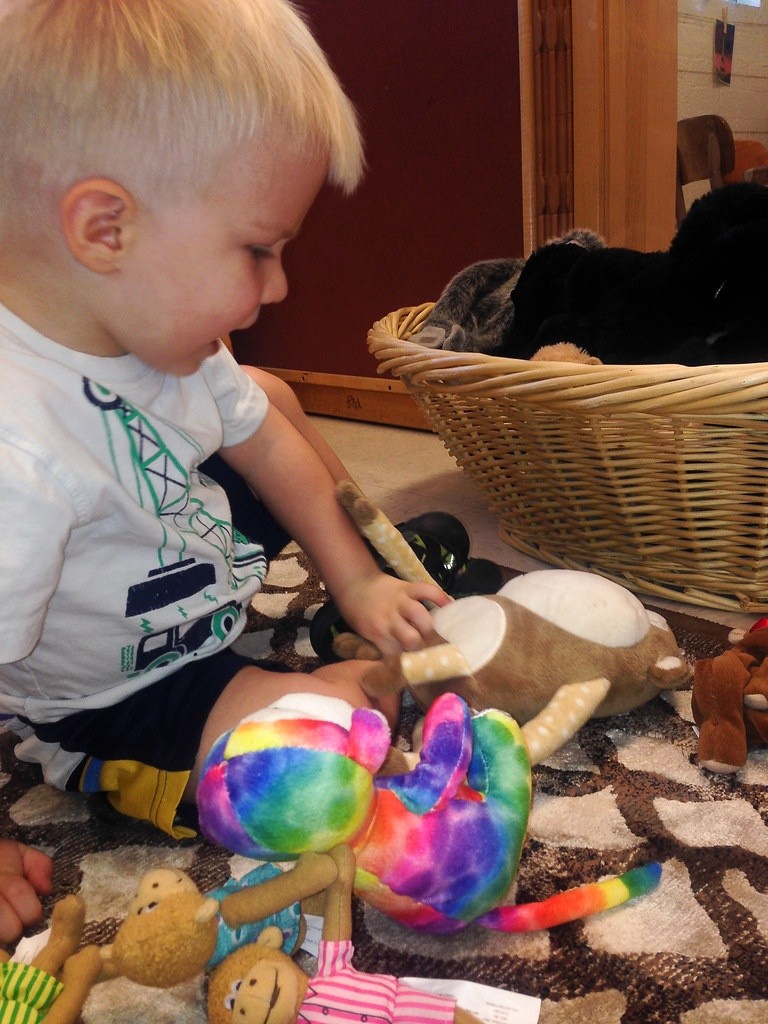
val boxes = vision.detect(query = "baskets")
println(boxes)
[368,303,768,613]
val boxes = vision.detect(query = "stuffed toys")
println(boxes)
[333,477,691,723]
[1,844,628,1024]
[693,618,768,773]
[197,690,662,936]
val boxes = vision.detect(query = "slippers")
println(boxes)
[310,511,505,665]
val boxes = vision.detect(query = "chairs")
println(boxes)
[676,114,736,230]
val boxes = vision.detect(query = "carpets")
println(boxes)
[0,557,768,1024]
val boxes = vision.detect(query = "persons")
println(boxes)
[1,0,453,946]
